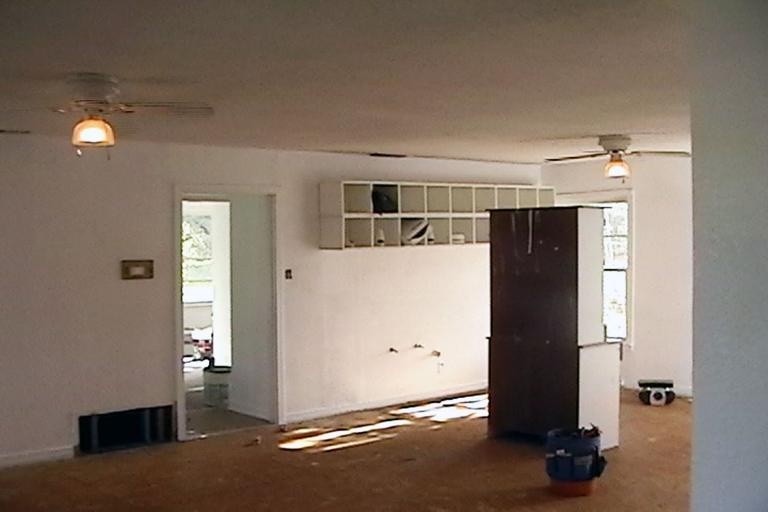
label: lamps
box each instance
[602,154,632,178]
[71,116,116,160]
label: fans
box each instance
[546,135,691,162]
[1,76,216,120]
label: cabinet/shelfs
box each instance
[320,178,553,249]
[485,205,620,452]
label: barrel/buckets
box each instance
[203,365,232,407]
